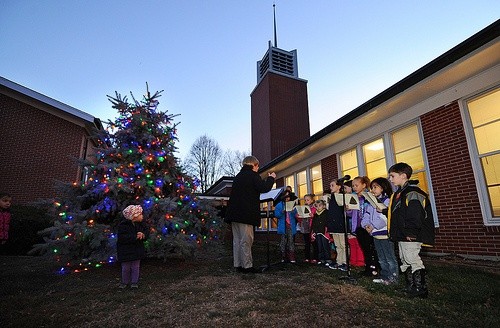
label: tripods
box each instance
[257,187,284,272]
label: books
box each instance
[334,193,360,210]
[286,198,298,211]
[360,192,387,209]
[295,205,311,218]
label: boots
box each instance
[403,268,428,299]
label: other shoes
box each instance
[236,266,255,273]
[374,278,391,285]
[329,263,366,272]
[131,284,138,291]
[116,285,127,293]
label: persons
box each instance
[274,176,400,286]
[376,163,435,299]
[224,156,276,274]
[116,205,150,290]
[0,192,13,245]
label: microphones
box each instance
[337,174,351,184]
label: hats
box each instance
[122,205,142,220]
